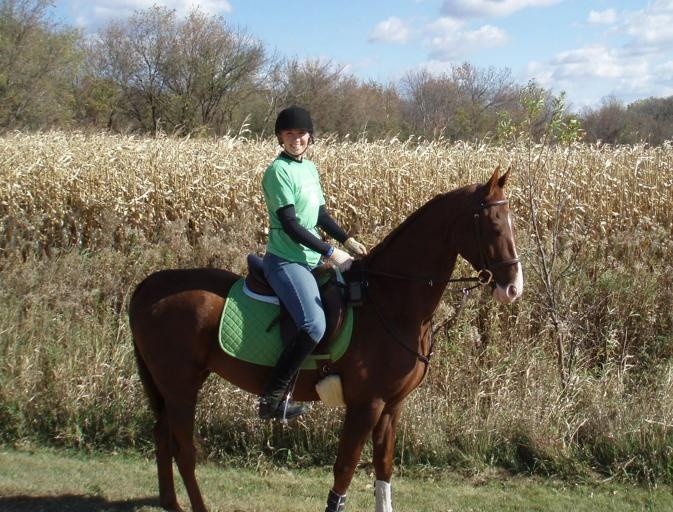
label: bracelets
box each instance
[325,246,334,258]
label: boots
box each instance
[260,329,319,418]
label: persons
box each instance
[257,106,368,420]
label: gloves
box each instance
[344,237,367,255]
[328,248,353,274]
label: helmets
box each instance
[275,107,312,136]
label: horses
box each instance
[126,166,524,512]
